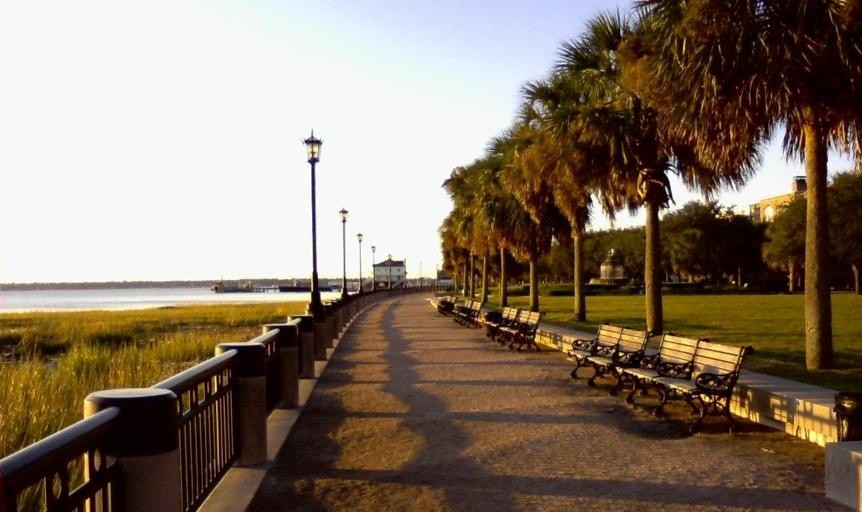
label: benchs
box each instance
[437,295,545,353]
[566,322,754,432]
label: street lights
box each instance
[371,246,375,290]
[356,232,364,293]
[304,129,322,309]
[388,253,391,290]
[339,208,348,295]
